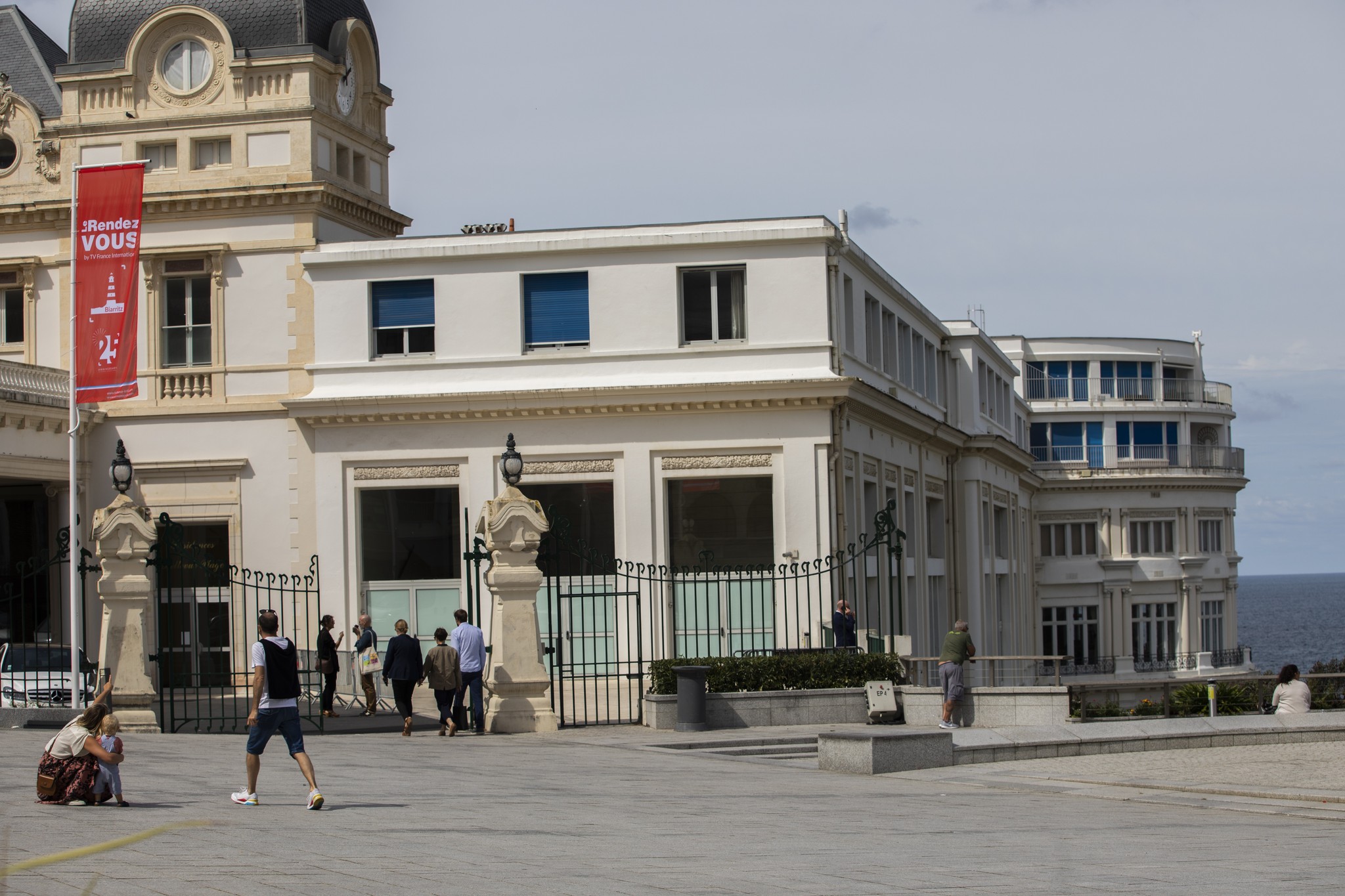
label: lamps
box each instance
[108,439,135,494]
[499,433,524,486]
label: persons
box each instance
[353,614,377,717]
[381,619,424,736]
[938,619,976,728]
[37,674,124,804]
[316,615,344,715]
[419,628,463,737]
[450,608,487,735]
[89,714,130,808]
[832,600,859,654]
[1272,664,1312,715]
[230,609,325,810]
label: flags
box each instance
[75,164,139,403]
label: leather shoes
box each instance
[449,723,457,737]
[439,731,446,736]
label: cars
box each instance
[0,642,97,709]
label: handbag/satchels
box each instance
[315,657,334,674]
[37,773,57,796]
[358,646,383,675]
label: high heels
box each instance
[319,709,326,716]
[325,710,340,718]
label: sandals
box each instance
[404,717,412,734]
[93,800,101,806]
[117,801,130,807]
[402,731,411,736]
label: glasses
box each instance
[259,609,278,619]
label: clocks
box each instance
[337,45,356,117]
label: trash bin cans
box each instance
[670,665,712,732]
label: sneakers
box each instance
[307,788,324,810]
[446,725,451,730]
[939,720,944,727]
[64,798,86,806]
[470,727,485,735]
[359,711,376,717]
[231,786,259,805]
[941,720,959,729]
